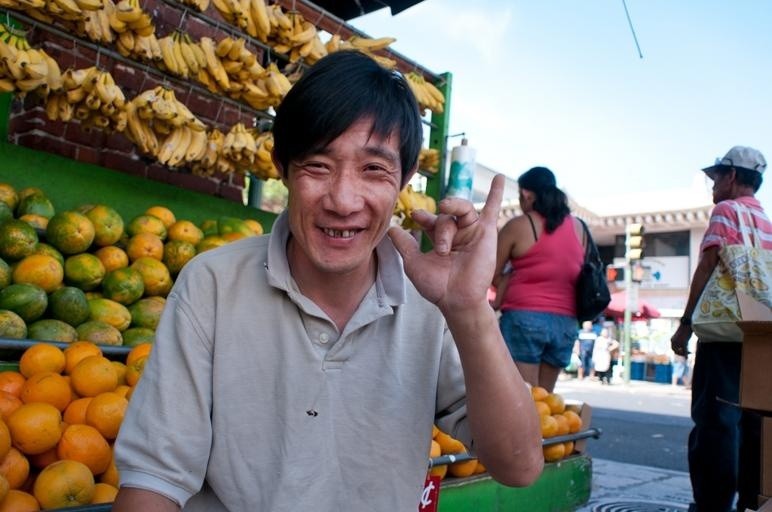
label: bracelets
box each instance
[679,316,692,326]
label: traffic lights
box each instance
[625,222,644,260]
[606,267,624,282]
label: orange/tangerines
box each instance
[524,380,584,463]
[0,183,263,346]
[429,424,486,484]
[0,341,159,512]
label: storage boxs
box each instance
[736,288,772,512]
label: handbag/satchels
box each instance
[692,243,772,342]
[576,262,611,321]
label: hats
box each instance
[702,145,766,180]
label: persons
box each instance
[489,258,514,311]
[672,355,690,387]
[489,166,591,393]
[671,145,772,511]
[591,326,620,386]
[111,49,546,512]
[576,320,597,384]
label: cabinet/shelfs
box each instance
[0,0,603,512]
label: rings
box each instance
[677,347,683,351]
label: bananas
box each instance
[186,1,446,117]
[0,10,281,180]
[418,149,441,175]
[0,0,298,110]
[396,184,439,224]
